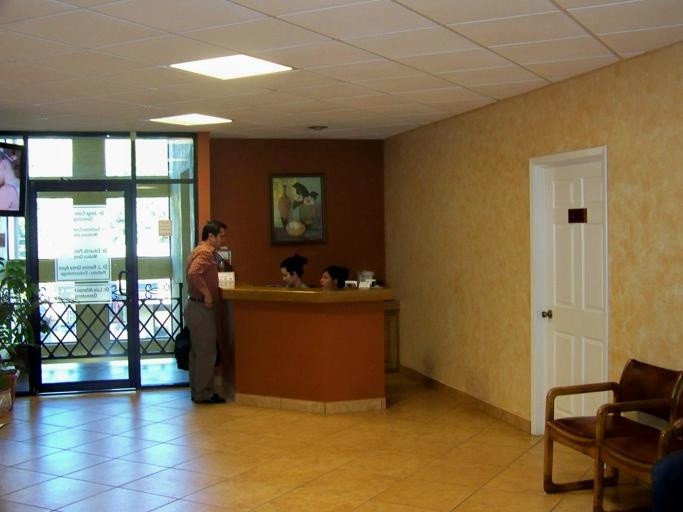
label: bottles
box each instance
[278,185,291,230]
[218,259,225,272]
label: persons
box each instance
[181,218,228,405]
[276,253,310,290]
[1,148,20,211]
[318,265,350,291]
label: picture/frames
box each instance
[271,172,327,246]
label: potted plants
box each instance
[0,258,53,420]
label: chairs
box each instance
[542,358,683,512]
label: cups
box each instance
[362,271,374,281]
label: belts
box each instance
[187,296,203,302]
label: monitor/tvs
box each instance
[0,143,26,216]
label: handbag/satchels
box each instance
[174,327,221,370]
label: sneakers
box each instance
[192,393,225,403]
